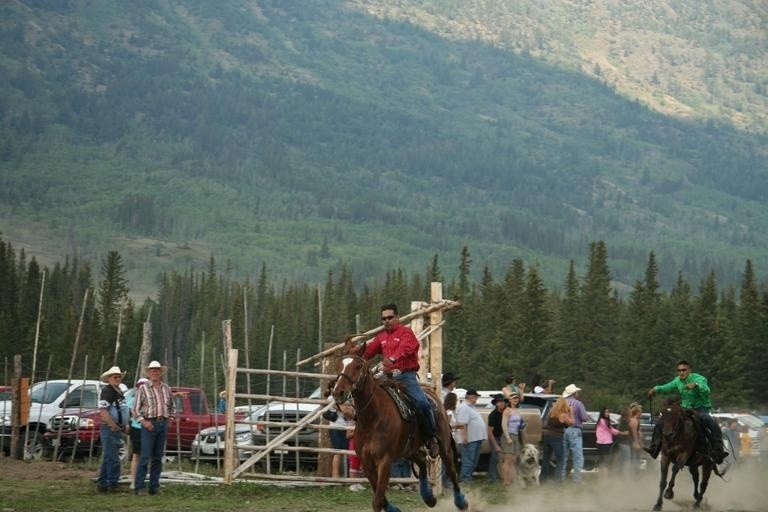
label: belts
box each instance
[146,416,166,422]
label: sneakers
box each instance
[428,439,439,458]
[641,447,658,459]
[358,483,366,489]
[350,485,359,492]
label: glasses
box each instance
[677,369,687,372]
[382,315,394,321]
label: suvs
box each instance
[710,413,767,458]
[0,380,133,462]
[252,385,322,473]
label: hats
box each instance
[562,384,581,397]
[136,378,149,386]
[509,392,519,398]
[100,366,126,383]
[442,372,460,386]
[492,394,508,405]
[143,361,168,374]
[466,388,481,396]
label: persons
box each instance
[219,391,228,414]
[594,407,629,481]
[529,373,555,394]
[128,378,150,490]
[539,398,576,484]
[500,392,529,490]
[642,360,729,464]
[444,392,464,473]
[457,389,486,485]
[488,394,506,485]
[346,413,366,493]
[757,422,768,478]
[363,304,439,458]
[502,376,526,409]
[740,425,756,464]
[97,366,130,493]
[133,361,177,497]
[324,380,356,478]
[615,408,632,474]
[561,384,595,487]
[440,372,460,405]
[725,420,739,465]
[629,406,643,473]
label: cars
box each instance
[190,402,284,461]
[487,393,620,470]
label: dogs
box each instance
[517,444,542,488]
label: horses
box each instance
[332,336,469,512]
[652,397,723,512]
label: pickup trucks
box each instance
[45,388,248,464]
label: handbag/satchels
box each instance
[322,404,338,421]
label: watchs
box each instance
[694,382,698,388]
[388,356,396,363]
